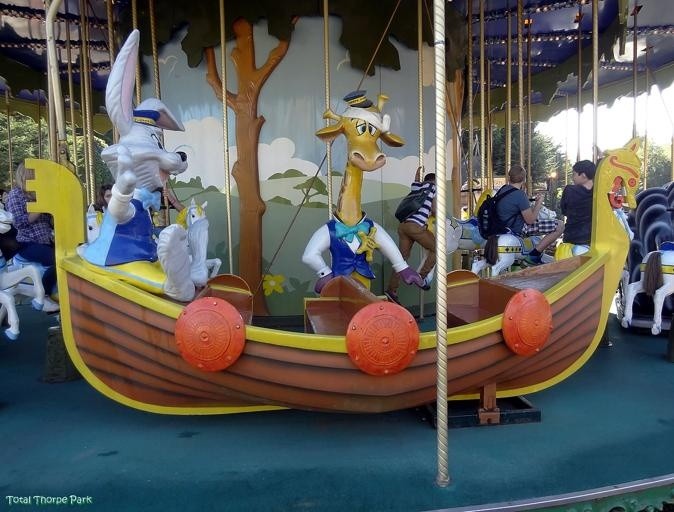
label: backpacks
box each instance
[478,184,519,240]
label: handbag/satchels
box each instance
[0,227,21,261]
[395,187,428,223]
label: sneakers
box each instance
[414,273,431,291]
[383,287,401,305]
[521,252,545,267]
[32,294,59,312]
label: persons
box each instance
[381,164,441,306]
[492,165,562,264]
[9,162,60,314]
[83,185,113,212]
[560,160,596,246]
